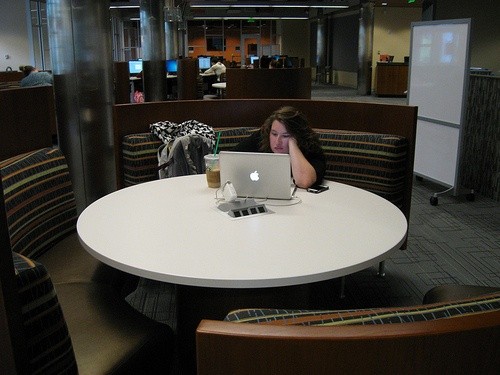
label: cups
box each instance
[204,154,220,188]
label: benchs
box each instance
[122,127,407,207]
[0,145,157,375]
[221,283,500,326]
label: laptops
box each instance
[219,150,296,200]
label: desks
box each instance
[376,61,409,98]
[130,72,226,99]
[76,174,408,300]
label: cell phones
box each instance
[307,185,329,194]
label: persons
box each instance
[268,59,282,68]
[20,65,53,87]
[204,57,227,94]
[235,106,326,189]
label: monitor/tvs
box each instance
[251,56,259,64]
[198,55,212,72]
[166,60,178,75]
[129,59,143,76]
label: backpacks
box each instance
[158,135,214,179]
